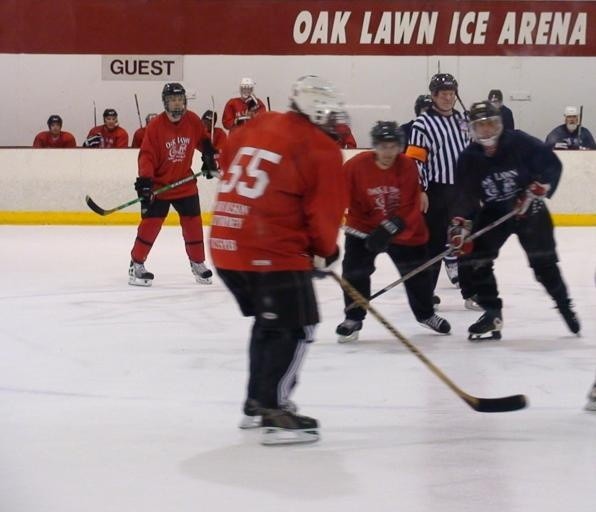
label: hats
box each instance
[563,106,579,116]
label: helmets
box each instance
[369,121,408,156]
[240,77,256,99]
[203,110,217,126]
[47,115,62,128]
[162,82,187,118]
[411,73,503,139]
[103,109,118,125]
[288,75,352,135]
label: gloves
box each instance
[247,96,260,112]
[365,213,405,255]
[201,158,217,179]
[234,116,250,125]
[515,181,551,219]
[447,216,472,257]
[134,177,153,204]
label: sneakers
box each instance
[129,260,154,279]
[336,319,362,336]
[445,262,458,284]
[468,309,503,332]
[420,314,450,333]
[243,398,318,429]
[190,260,212,278]
[556,298,580,333]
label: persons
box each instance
[545,106,595,150]
[84,110,129,147]
[405,95,432,140]
[209,77,345,430]
[488,90,514,129]
[409,75,502,310]
[132,78,358,150]
[445,100,581,341]
[129,83,220,279]
[33,115,75,148]
[335,122,450,333]
